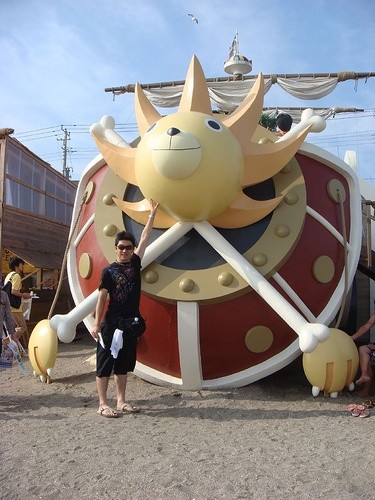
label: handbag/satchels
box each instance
[1,273,22,309]
[119,315,147,341]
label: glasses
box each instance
[116,244,134,250]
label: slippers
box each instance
[345,399,375,418]
[97,403,141,418]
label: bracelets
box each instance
[149,215,155,217]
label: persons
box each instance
[0,257,32,359]
[91,198,159,417]
[350,299,375,397]
[277,113,293,136]
